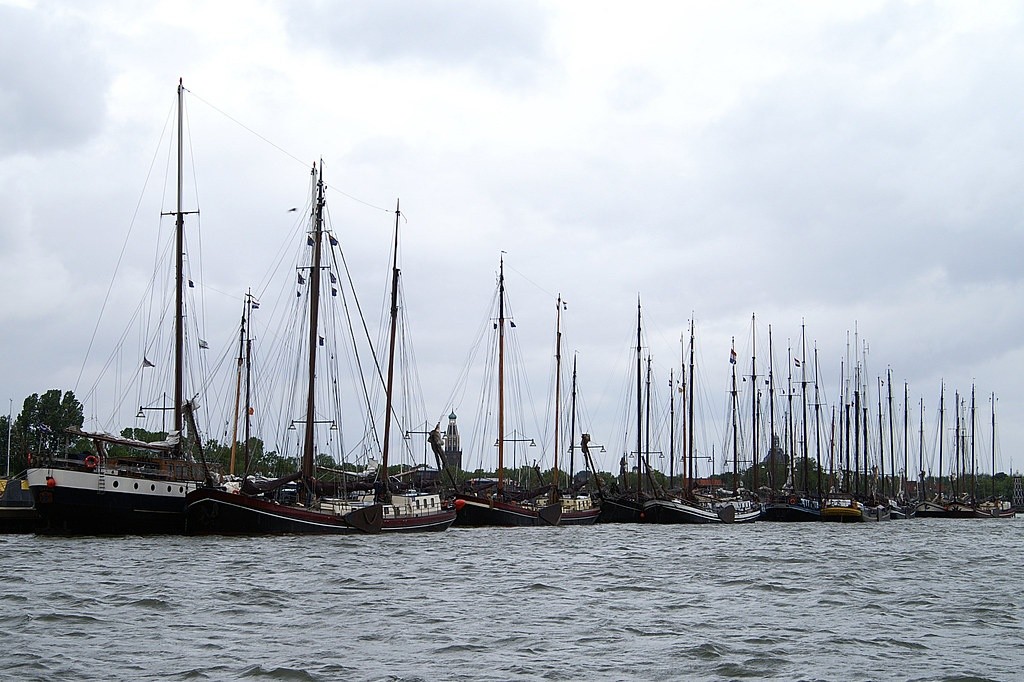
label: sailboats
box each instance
[0,76,1017,538]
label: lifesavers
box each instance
[84,455,97,469]
[790,499,795,505]
